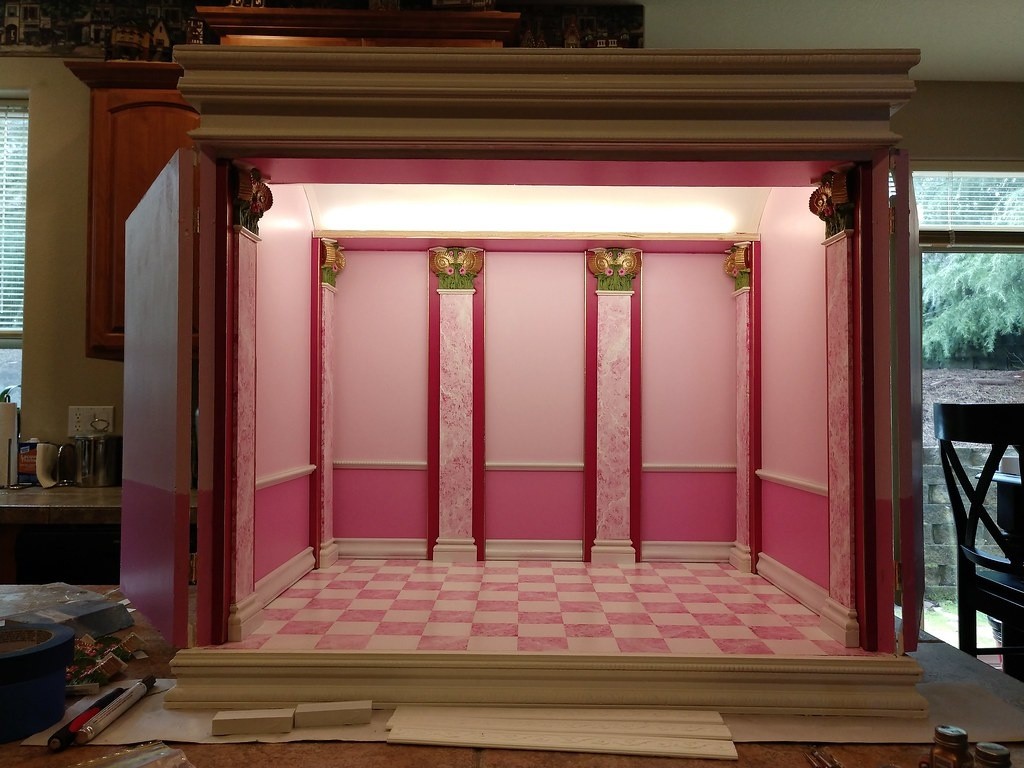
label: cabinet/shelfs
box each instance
[64,6,522,362]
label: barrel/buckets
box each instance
[72,418,122,486]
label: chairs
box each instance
[933,402,1024,680]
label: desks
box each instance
[0,489,199,584]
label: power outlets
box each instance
[66,404,113,439]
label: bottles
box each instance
[930,725,973,768]
[973,742,1011,768]
